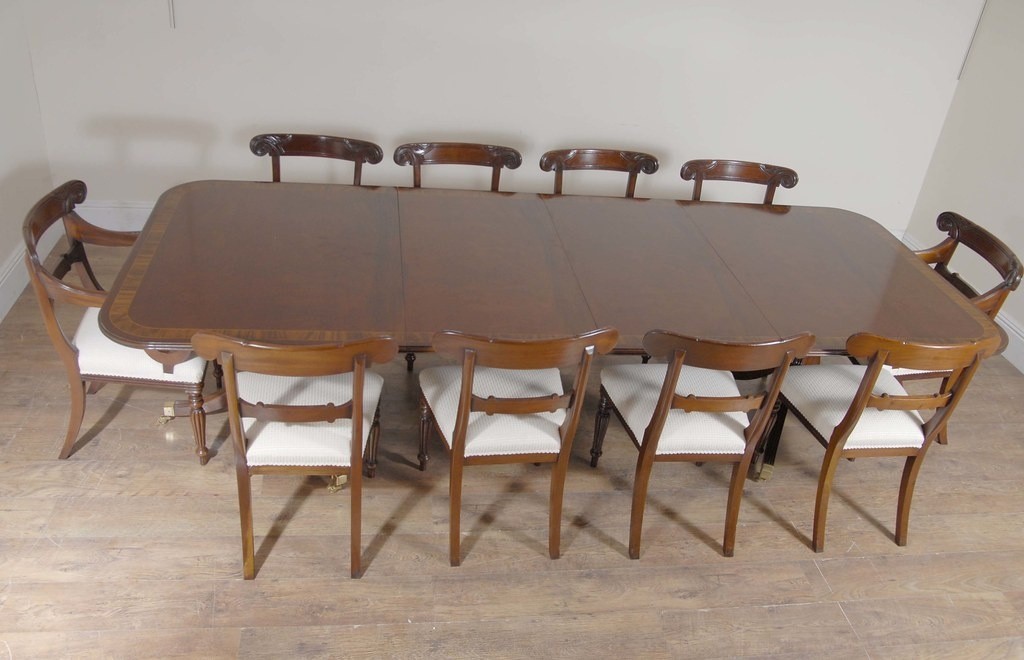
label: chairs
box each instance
[887,212,1023,445]
[207,134,999,580]
[23,180,224,466]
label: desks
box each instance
[97,180,1008,481]
